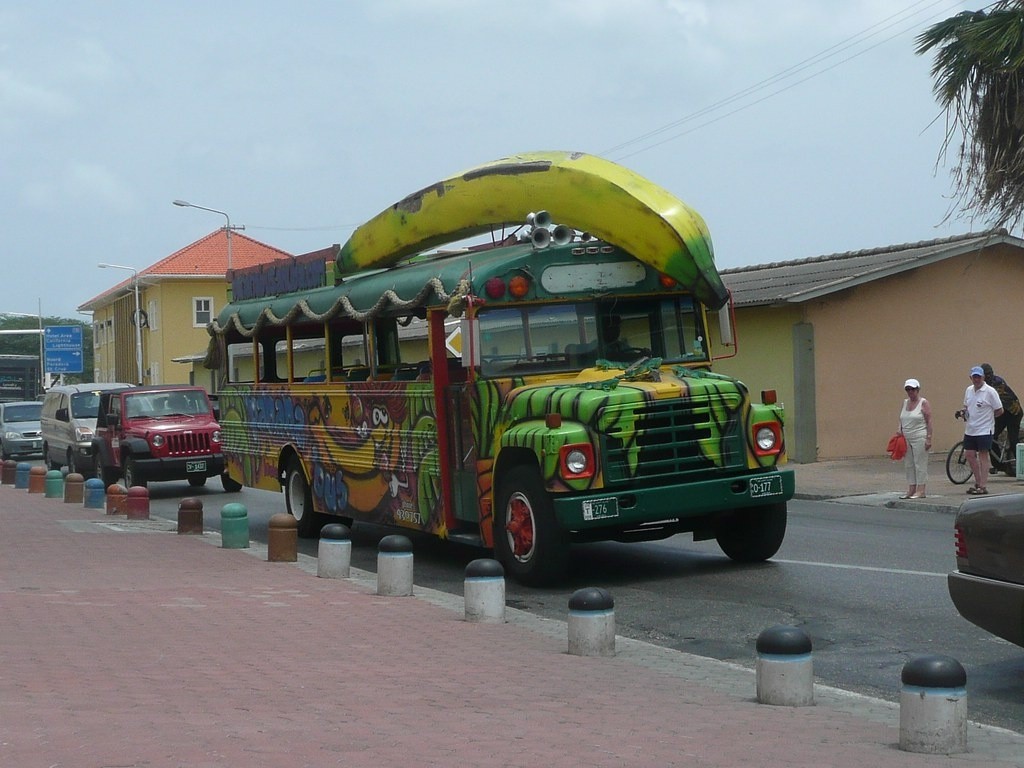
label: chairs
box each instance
[128,399,145,416]
[167,397,187,411]
[563,337,634,369]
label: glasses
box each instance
[905,387,917,391]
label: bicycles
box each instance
[945,408,1024,486]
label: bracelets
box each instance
[928,435,931,438]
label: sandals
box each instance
[966,484,988,494]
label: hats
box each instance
[971,367,984,376]
[905,379,920,388]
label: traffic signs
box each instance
[44,325,84,374]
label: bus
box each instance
[215,150,796,583]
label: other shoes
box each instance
[898,494,914,499]
[909,494,926,498]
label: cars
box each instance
[0,401,44,459]
[947,493,1024,648]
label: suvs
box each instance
[92,384,244,493]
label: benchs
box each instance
[489,355,548,373]
[392,362,462,381]
[345,363,412,381]
[305,364,366,382]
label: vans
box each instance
[40,383,138,475]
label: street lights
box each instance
[172,199,233,270]
[97,262,143,386]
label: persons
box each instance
[585,310,652,360]
[898,378,932,499]
[964,363,1024,494]
